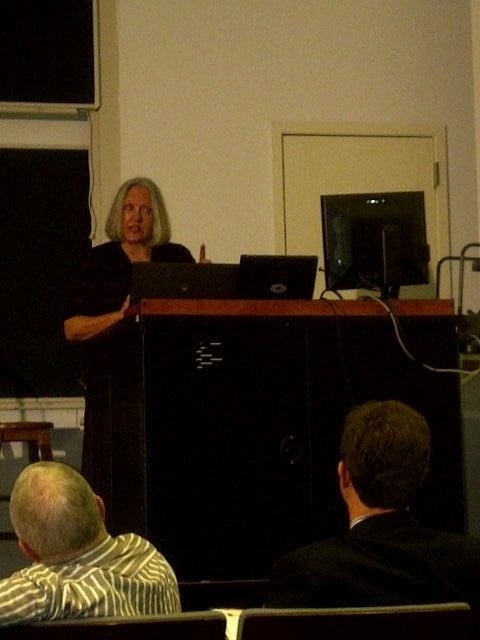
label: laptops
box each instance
[236,255,318,300]
[130,262,240,307]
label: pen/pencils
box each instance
[200,243,205,263]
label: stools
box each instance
[0,422,54,540]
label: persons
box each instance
[262,399,480,612]
[63,178,214,342]
[1,459,183,629]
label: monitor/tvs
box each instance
[321,191,428,299]
